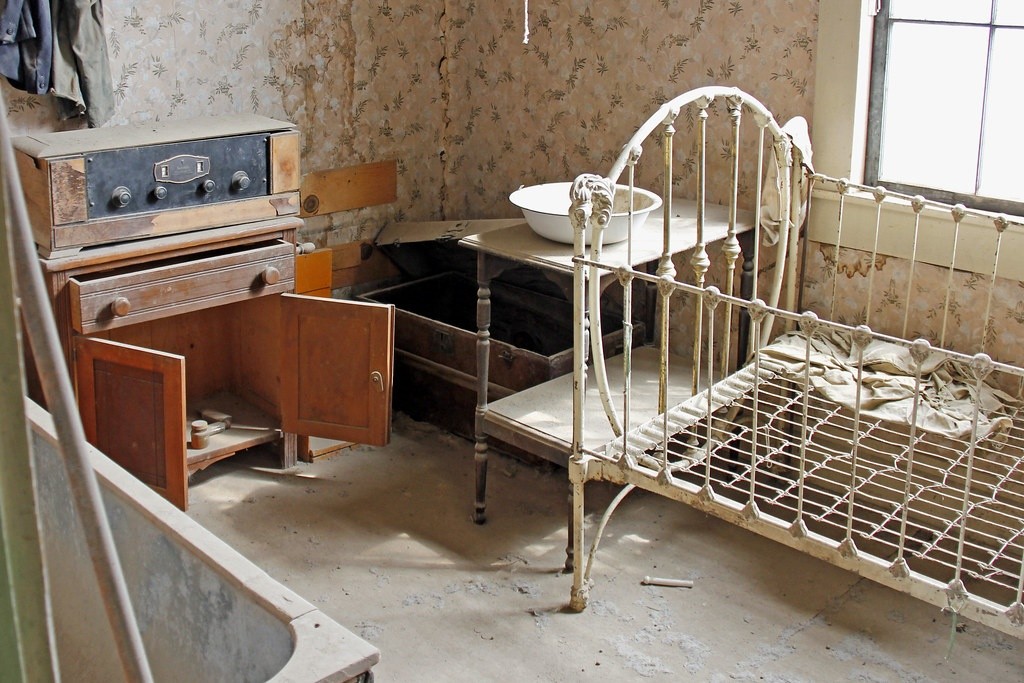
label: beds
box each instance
[566,86,1024,639]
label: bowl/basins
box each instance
[510,184,663,244]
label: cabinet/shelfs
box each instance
[17,216,397,513]
[457,195,761,571]
[351,270,646,468]
